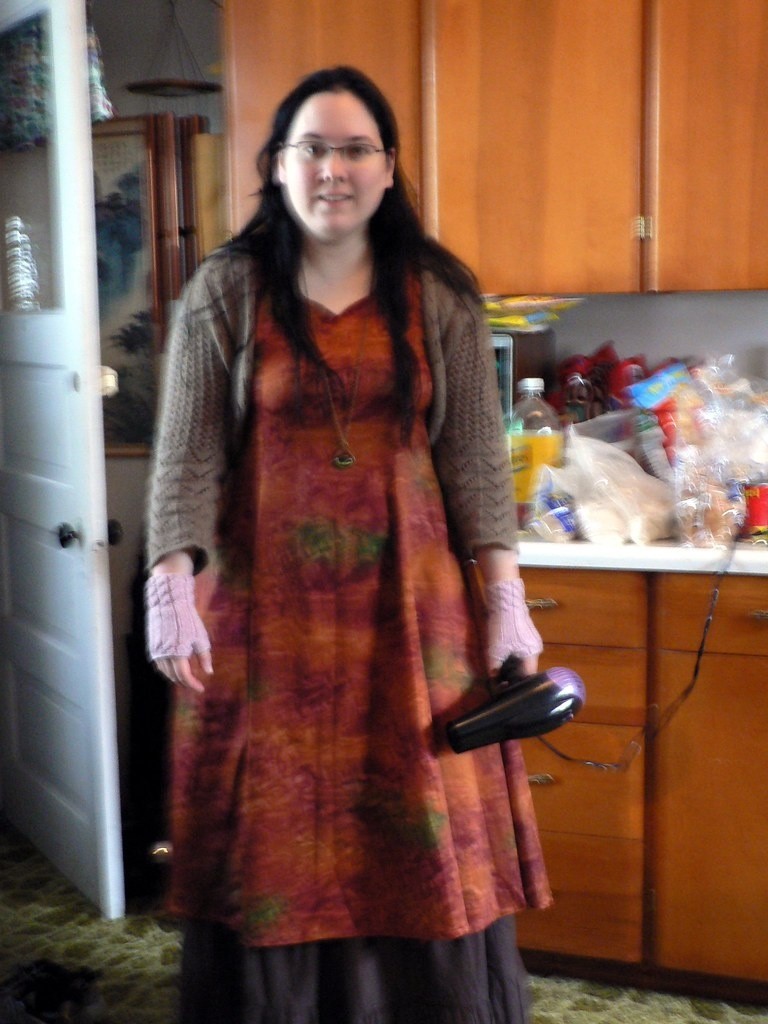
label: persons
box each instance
[147,65,542,1023]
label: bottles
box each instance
[507,378,558,533]
[525,491,581,543]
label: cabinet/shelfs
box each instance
[648,573,768,982]
[515,564,648,963]
[421,0,768,296]
[224,0,421,243]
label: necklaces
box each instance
[294,243,377,470]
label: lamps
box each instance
[124,0,222,98]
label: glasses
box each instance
[280,141,389,164]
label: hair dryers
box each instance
[441,655,585,755]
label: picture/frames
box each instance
[90,111,185,461]
